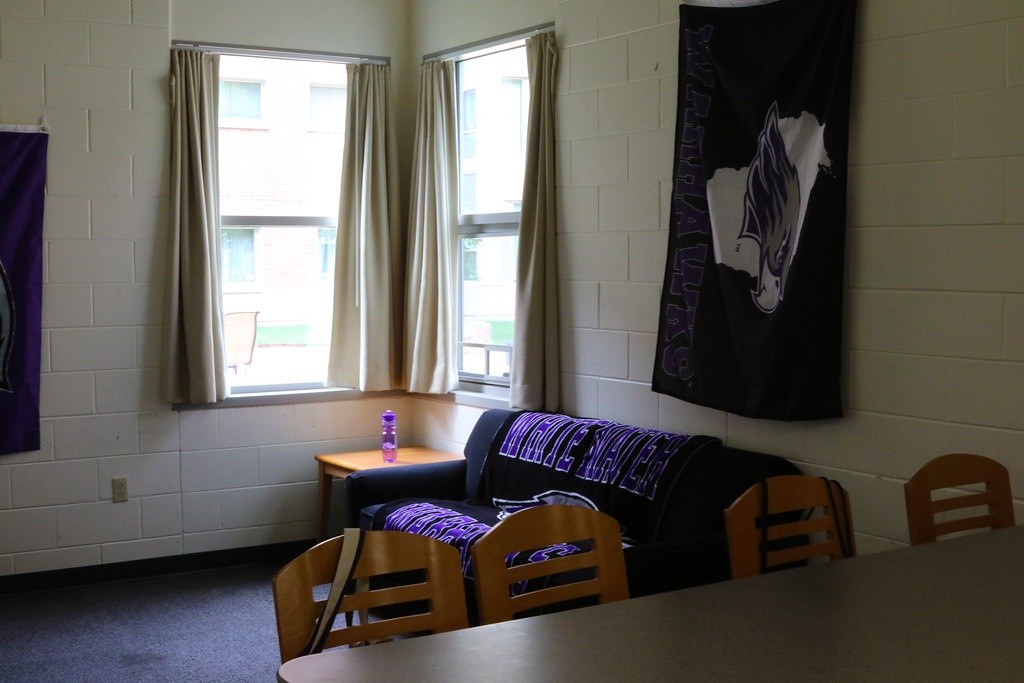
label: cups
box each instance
[382,409,397,462]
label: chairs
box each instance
[724,474,858,581]
[470,505,631,633]
[272,528,468,663]
[904,453,1015,546]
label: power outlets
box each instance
[112,477,128,502]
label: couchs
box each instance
[342,409,811,635]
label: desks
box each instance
[315,445,465,544]
[275,522,1023,683]
